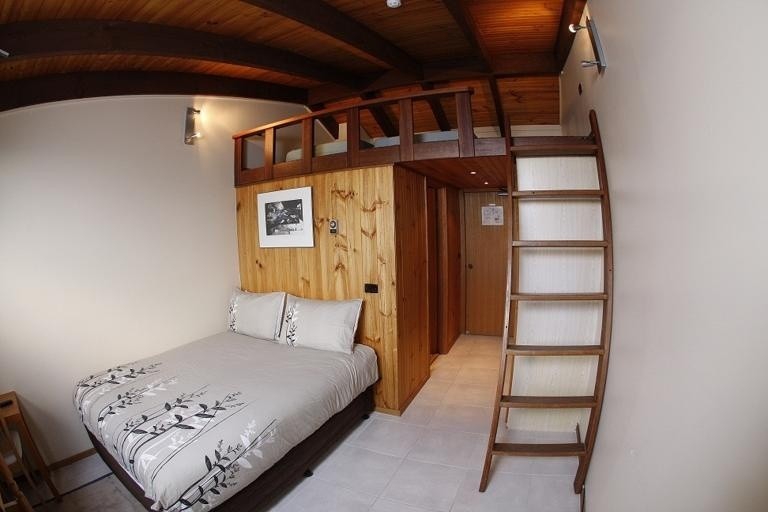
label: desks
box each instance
[0,391,64,504]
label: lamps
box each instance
[568,16,606,75]
[184,107,201,145]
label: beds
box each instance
[77,331,374,512]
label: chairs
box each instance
[0,415,45,509]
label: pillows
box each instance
[279,293,364,355]
[226,288,286,344]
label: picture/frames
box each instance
[256,186,315,249]
